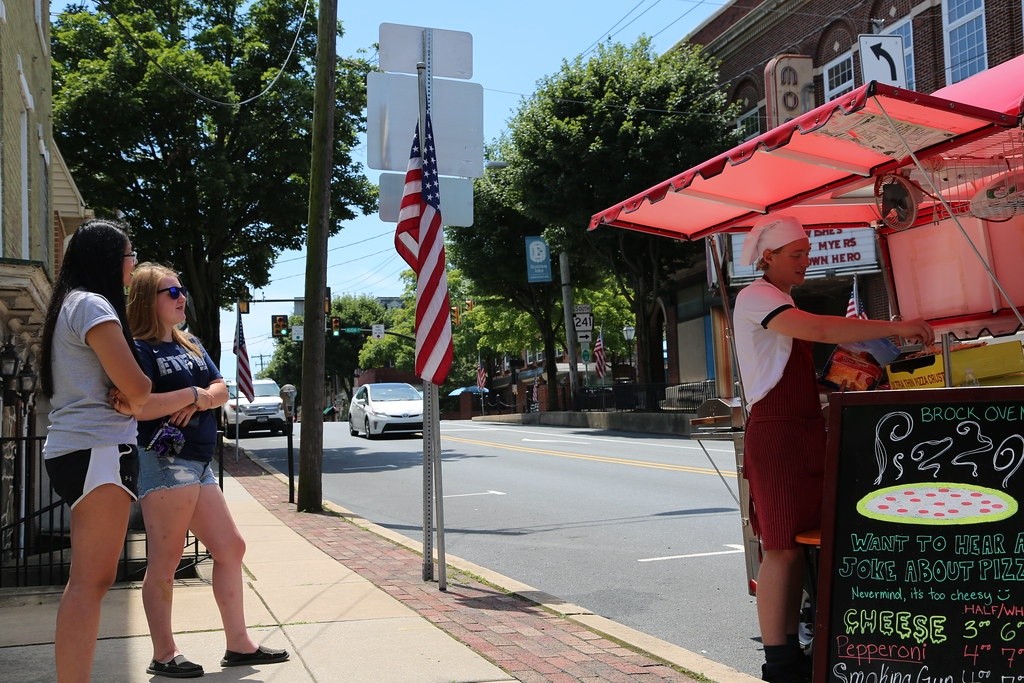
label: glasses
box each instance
[124,251,137,264]
[157,286,187,300]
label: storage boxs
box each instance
[885,340,1024,391]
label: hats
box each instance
[741,214,808,266]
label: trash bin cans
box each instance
[612,364,637,412]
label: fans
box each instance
[873,168,924,232]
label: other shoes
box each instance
[760,648,812,683]
[146,654,205,678]
[222,644,289,667]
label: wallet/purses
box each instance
[152,418,185,457]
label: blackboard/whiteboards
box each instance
[808,384,1023,683]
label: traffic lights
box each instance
[332,315,340,336]
[272,314,288,338]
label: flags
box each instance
[477,366,487,389]
[532,379,538,403]
[846,286,868,319]
[593,334,607,379]
[233,306,254,403]
[391,81,454,386]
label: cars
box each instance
[217,376,286,440]
[346,383,424,442]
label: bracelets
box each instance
[191,386,199,404]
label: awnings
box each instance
[322,406,333,414]
[588,79,1024,424]
[448,385,489,396]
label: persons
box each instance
[127,261,291,678]
[38,219,152,683]
[732,212,935,683]
